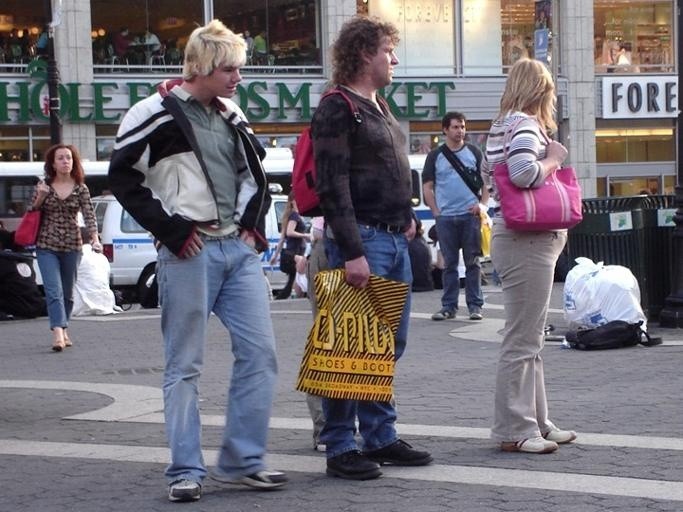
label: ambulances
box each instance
[83,181,311,307]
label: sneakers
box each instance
[208,469,290,488]
[432,304,456,320]
[52,340,72,350]
[168,479,201,502]
[500,426,577,454]
[469,303,482,320]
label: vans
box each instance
[402,153,494,269]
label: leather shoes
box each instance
[317,439,434,481]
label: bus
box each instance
[0,147,295,232]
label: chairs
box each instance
[8,42,276,73]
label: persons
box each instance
[70,226,123,318]
[421,108,489,321]
[29,144,105,351]
[535,9,548,29]
[474,57,582,455]
[106,18,286,501]
[1,18,48,73]
[243,28,315,73]
[605,45,630,73]
[270,14,435,483]
[94,26,187,72]
[410,220,467,292]
[269,191,325,300]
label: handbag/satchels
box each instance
[494,164,583,232]
[14,210,41,246]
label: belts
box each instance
[355,213,404,234]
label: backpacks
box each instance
[291,88,359,217]
[566,320,662,350]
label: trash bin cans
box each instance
[567,195,681,326]
